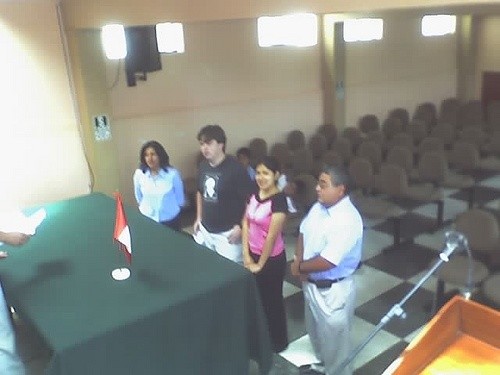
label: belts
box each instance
[308,277,346,284]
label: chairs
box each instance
[184,100,500,317]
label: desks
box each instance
[0,192,274,375]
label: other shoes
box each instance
[299,363,325,375]
[272,341,288,353]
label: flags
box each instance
[113,191,134,265]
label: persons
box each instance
[236,147,296,213]
[0,231,29,259]
[192,125,252,262]
[132,141,186,230]
[292,166,364,375]
[240,156,287,352]
